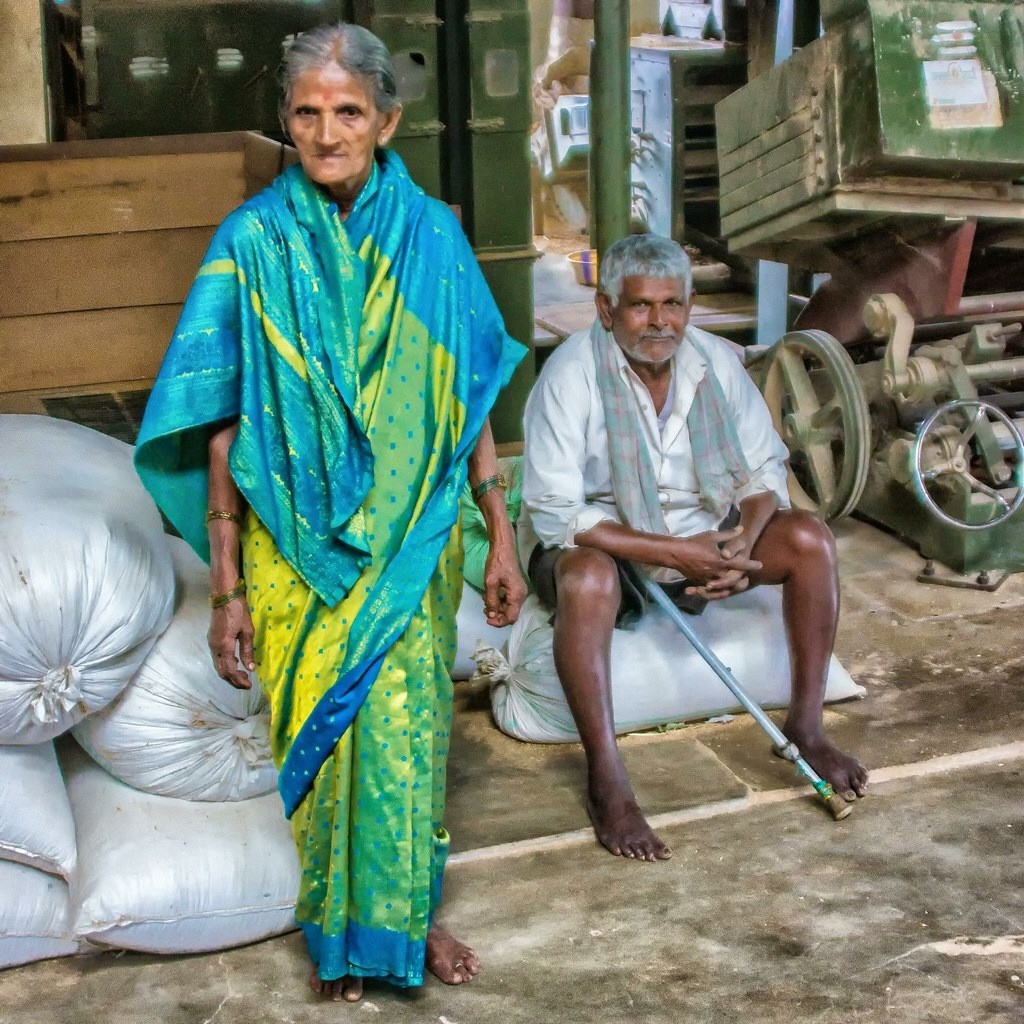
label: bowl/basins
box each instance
[566,249,597,286]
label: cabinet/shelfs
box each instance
[589,32,747,242]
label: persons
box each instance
[516,237,867,863]
[133,25,529,1002]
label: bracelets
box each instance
[208,577,247,608]
[202,511,244,527]
[470,475,508,505]
